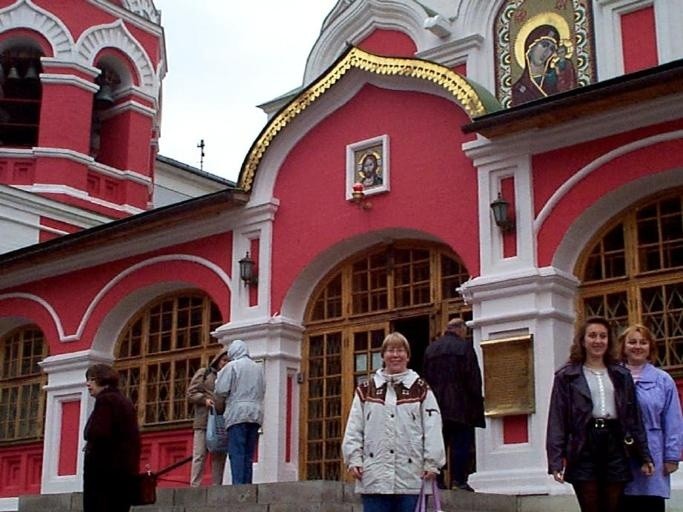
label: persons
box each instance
[182,350,229,486]
[513,22,558,104]
[214,338,265,483]
[543,315,654,510]
[338,330,446,509]
[423,316,486,492]
[357,153,382,188]
[615,321,682,511]
[555,46,578,95]
[81,364,141,512]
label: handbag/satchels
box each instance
[205,404,228,454]
[130,470,159,506]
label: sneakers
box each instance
[437,481,475,492]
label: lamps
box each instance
[489,191,517,236]
[238,251,257,287]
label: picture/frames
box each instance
[344,134,391,202]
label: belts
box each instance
[588,417,621,428]
